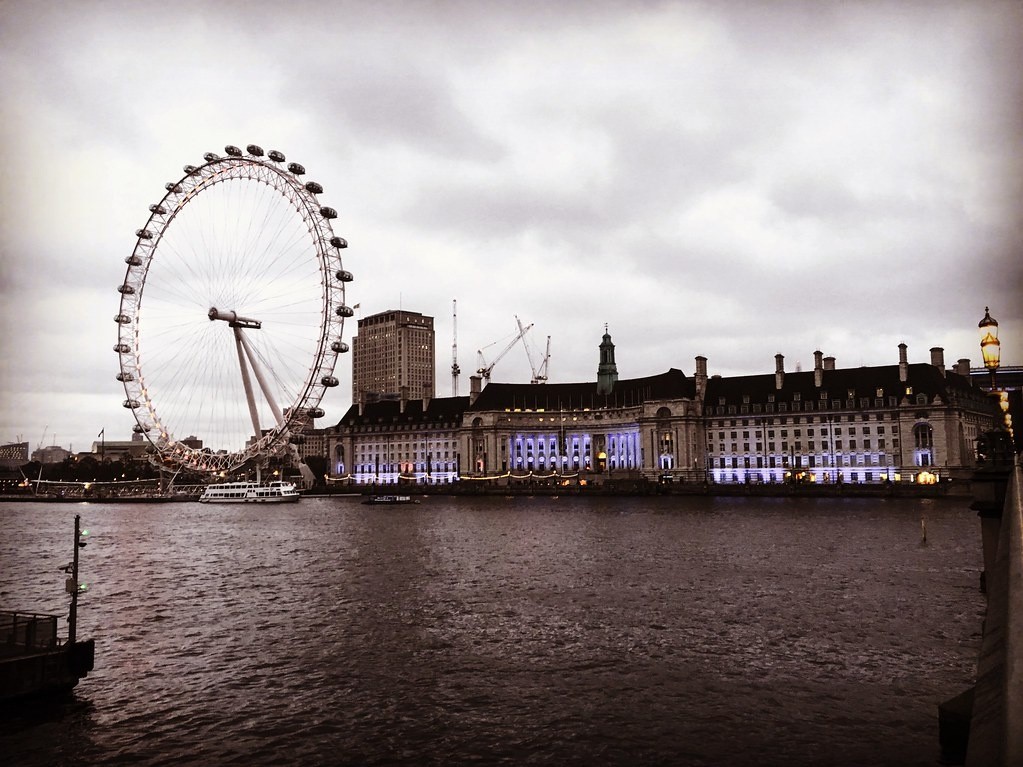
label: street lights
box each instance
[703,422,708,482]
[386,434,390,473]
[761,418,768,468]
[896,410,903,467]
[424,432,429,483]
[979,306,1003,430]
[828,416,835,484]
[999,386,1014,443]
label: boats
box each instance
[361,495,421,504]
[198,481,300,502]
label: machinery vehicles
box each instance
[451,300,551,397]
